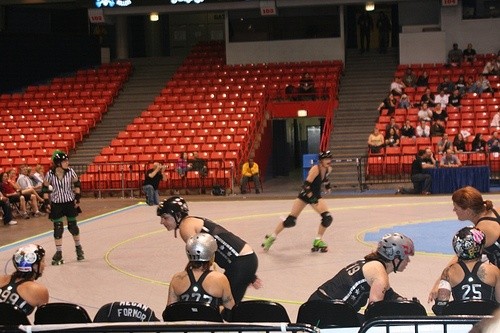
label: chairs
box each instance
[0,299,500,333]
[367,52,500,177]
[0,41,343,190]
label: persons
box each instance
[261,151,334,252]
[177,150,208,180]
[157,196,263,305]
[241,157,261,194]
[427,185,500,304]
[365,43,500,196]
[142,161,169,206]
[297,72,316,100]
[41,149,86,265]
[295,232,415,329]
[166,233,237,324]
[0,164,53,225]
[0,243,49,317]
[431,226,500,316]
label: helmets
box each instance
[379,233,414,259]
[12,244,46,274]
[451,227,486,260]
[185,233,218,262]
[157,196,188,217]
[319,150,332,160]
[53,153,70,165]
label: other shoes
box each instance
[8,220,17,225]
[40,205,51,213]
[39,199,51,204]
[22,213,29,218]
[147,200,153,206]
[34,210,45,217]
[256,189,260,194]
[423,190,431,195]
[241,190,246,193]
[395,188,403,194]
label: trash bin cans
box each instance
[303,153,320,180]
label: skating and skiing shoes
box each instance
[52,251,64,265]
[311,239,328,252]
[75,245,84,260]
[262,234,276,251]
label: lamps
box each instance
[365,0,375,11]
[150,0,159,21]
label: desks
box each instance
[423,165,489,194]
